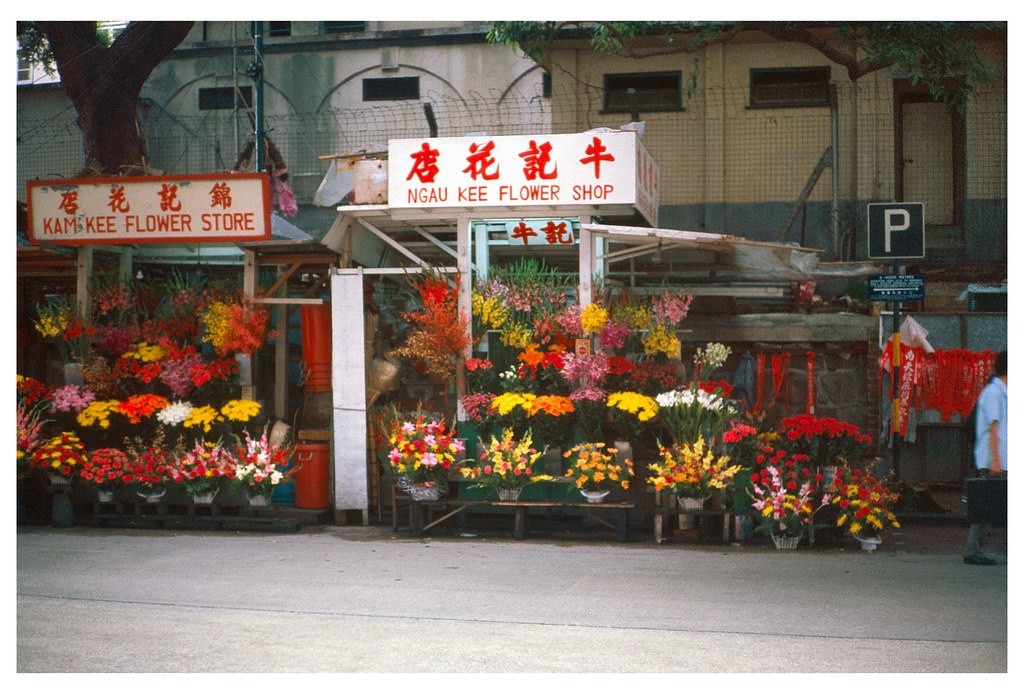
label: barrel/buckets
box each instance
[269,442,330,510]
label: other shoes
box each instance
[964,555,997,566]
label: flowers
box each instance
[16,276,894,533]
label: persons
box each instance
[961,351,1008,566]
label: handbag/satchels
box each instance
[962,472,1007,523]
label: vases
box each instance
[399,479,445,501]
[769,535,881,551]
[187,490,217,503]
[96,490,115,500]
[497,488,519,501]
[247,494,271,506]
[675,498,705,510]
[136,491,166,503]
[582,488,610,503]
[50,507,74,527]
[47,474,73,485]
